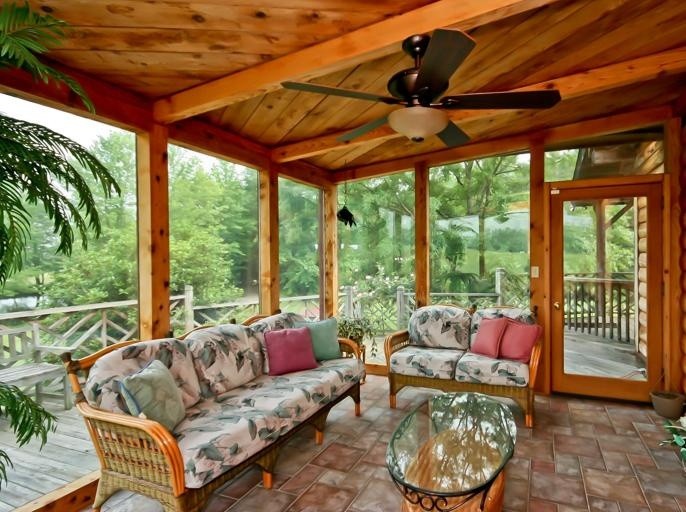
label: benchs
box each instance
[384,302,543,434]
[1,322,77,413]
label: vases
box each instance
[649,391,686,418]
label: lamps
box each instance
[385,95,448,144]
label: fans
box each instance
[281,28,561,149]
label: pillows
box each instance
[468,312,509,358]
[263,327,320,374]
[292,316,344,363]
[496,315,546,364]
[123,357,187,436]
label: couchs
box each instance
[61,311,367,512]
